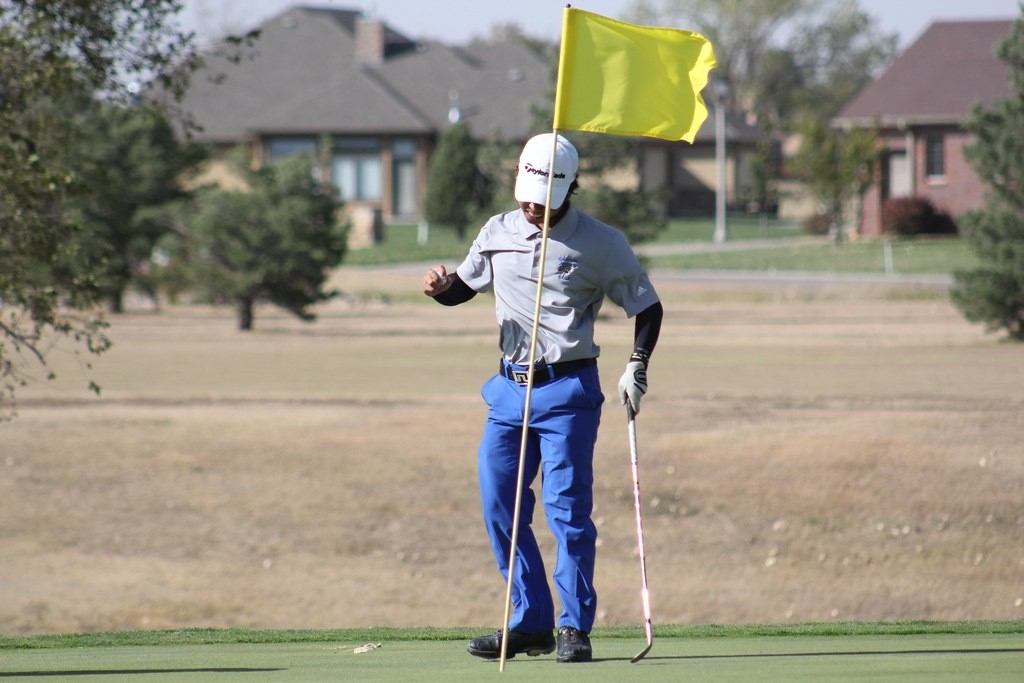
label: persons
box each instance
[423,133,664,663]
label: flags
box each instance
[553,5,717,144]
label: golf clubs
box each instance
[626,397,654,663]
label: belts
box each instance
[499,357,597,385]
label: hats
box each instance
[514,132,579,210]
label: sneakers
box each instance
[467,629,557,659]
[556,626,592,662]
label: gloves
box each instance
[618,362,648,416]
